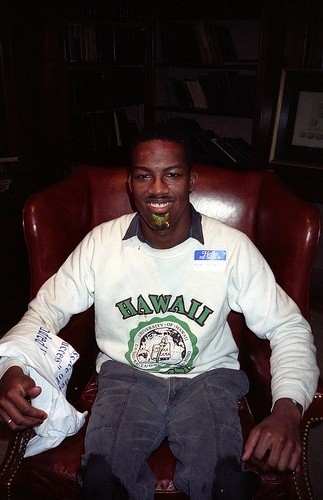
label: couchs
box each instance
[0,164,323,500]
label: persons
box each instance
[0,130,322,500]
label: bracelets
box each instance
[288,397,302,407]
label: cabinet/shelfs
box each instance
[147,0,288,171]
[37,0,160,177]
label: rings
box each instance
[6,418,13,426]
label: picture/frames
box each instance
[270,69,323,169]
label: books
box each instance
[65,0,262,166]
